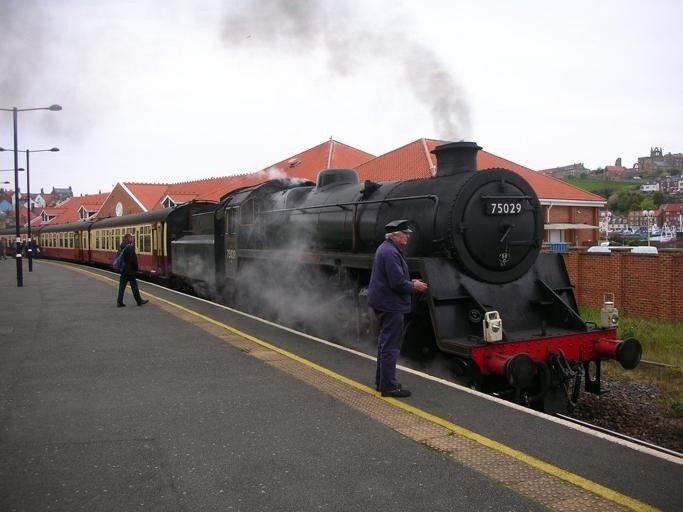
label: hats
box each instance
[125,233,136,238]
[384,220,414,233]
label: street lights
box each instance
[643,210,655,246]
[1,104,62,286]
[600,211,612,242]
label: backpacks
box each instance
[113,243,131,274]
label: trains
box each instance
[2,142,642,414]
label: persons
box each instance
[365,219,427,398]
[116,233,149,306]
[0,237,40,261]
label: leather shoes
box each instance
[137,300,149,306]
[117,303,126,307]
[377,381,412,398]
